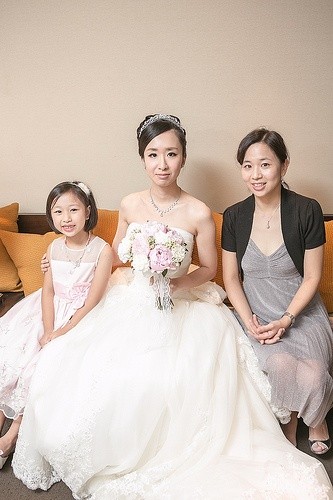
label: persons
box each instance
[222,128,333,454]
[0,181,114,470]
[10,114,333,500]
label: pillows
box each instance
[0,202,23,293]
[190,211,241,304]
[93,208,130,272]
[0,229,63,298]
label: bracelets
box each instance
[281,312,296,328]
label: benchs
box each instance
[0,214,333,325]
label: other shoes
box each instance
[0,456,8,469]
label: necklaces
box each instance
[149,187,182,217]
[66,231,90,273]
[267,206,279,229]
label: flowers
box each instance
[116,221,187,311]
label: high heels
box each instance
[307,420,331,456]
[283,421,299,450]
